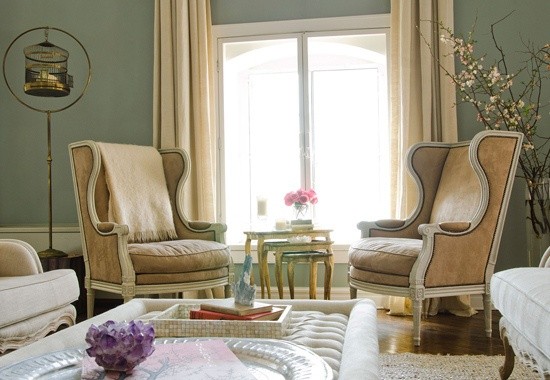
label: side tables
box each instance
[38,255,83,312]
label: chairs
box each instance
[348,131,524,347]
[67,138,232,321]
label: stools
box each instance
[272,251,333,300]
[260,240,335,298]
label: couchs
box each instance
[0,238,80,354]
[489,244,550,380]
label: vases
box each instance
[524,177,550,267]
[292,206,307,220]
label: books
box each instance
[188,304,286,323]
[202,296,272,317]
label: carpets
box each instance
[379,352,550,379]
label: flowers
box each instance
[284,189,319,212]
[416,6,549,238]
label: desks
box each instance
[9,296,379,379]
[244,229,334,302]
